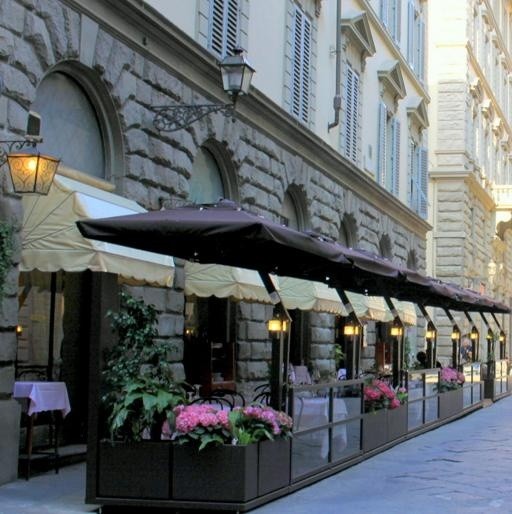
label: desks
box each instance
[14,381,72,481]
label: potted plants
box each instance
[97,295,195,500]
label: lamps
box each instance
[470,332,478,340]
[267,317,287,333]
[343,323,359,336]
[426,329,437,338]
[453,330,460,339]
[151,45,257,133]
[391,326,404,337]
[1,139,63,197]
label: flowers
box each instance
[274,408,294,434]
[160,400,278,451]
[364,380,407,409]
[439,367,465,392]
